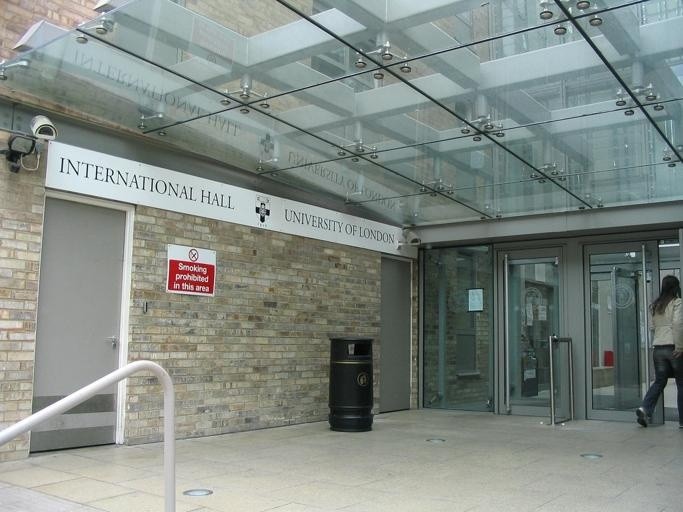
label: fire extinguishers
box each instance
[522,349,538,396]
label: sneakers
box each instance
[636,407,649,428]
[679,424,683,428]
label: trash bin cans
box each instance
[329,336,373,430]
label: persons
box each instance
[634,274,682,428]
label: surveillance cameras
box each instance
[30,115,59,141]
[406,232,421,245]
